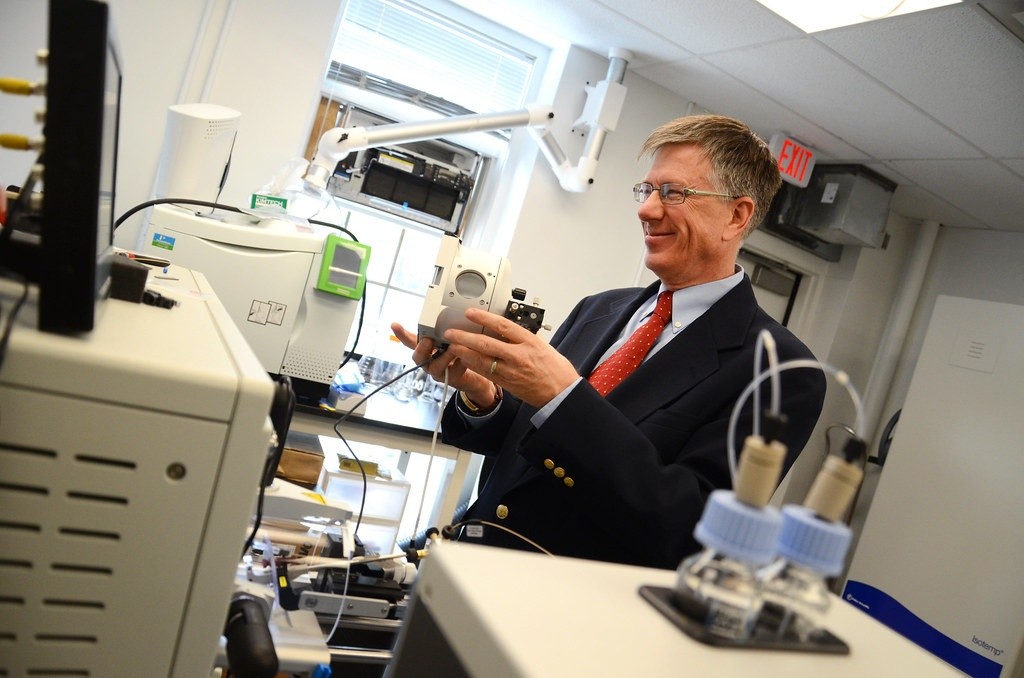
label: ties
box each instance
[586,290,675,399]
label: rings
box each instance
[489,358,498,375]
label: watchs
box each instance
[460,382,503,415]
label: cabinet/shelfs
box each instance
[241,373,471,678]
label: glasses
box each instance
[633,181,740,206]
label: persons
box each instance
[391,114,828,573]
